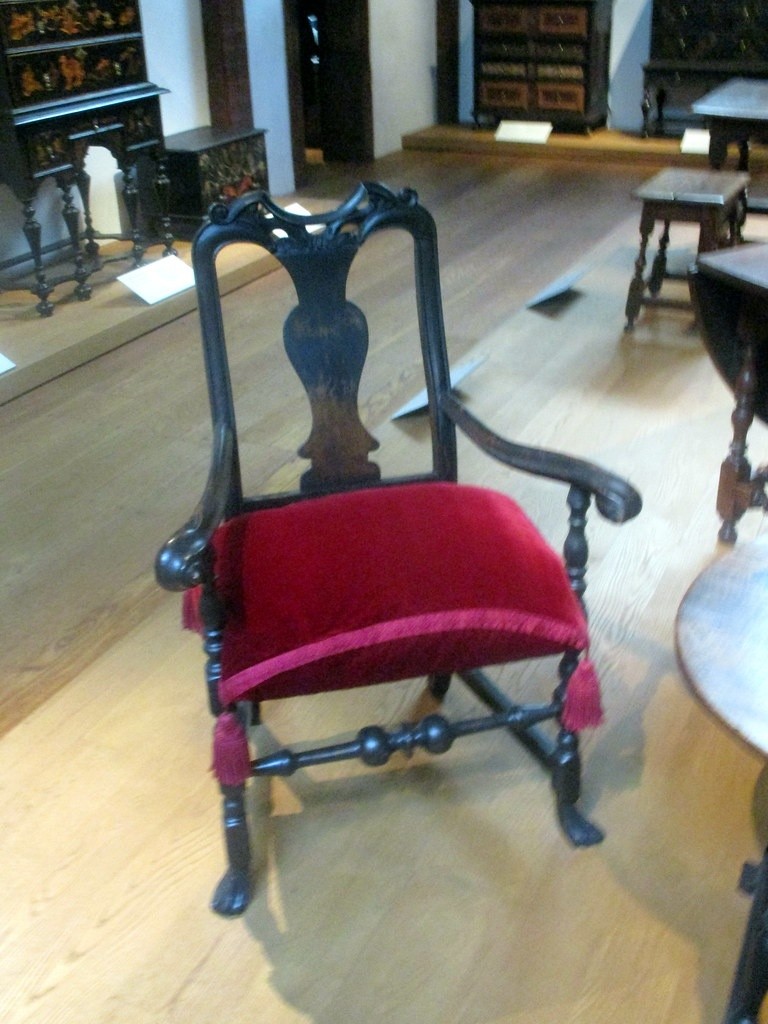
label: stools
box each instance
[624,166,752,337]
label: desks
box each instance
[691,242,768,543]
[690,78,768,214]
[675,530,768,1024]
[162,125,270,243]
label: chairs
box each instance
[155,180,642,917]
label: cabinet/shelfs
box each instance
[0,0,177,319]
[469,0,613,136]
[639,0,768,139]
